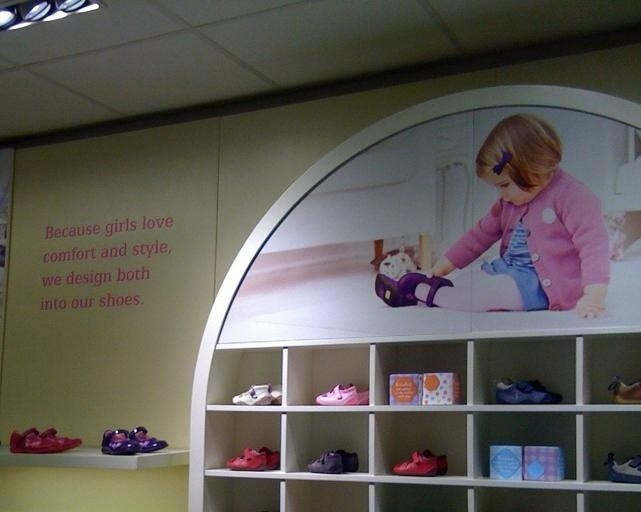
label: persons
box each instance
[374,112,611,318]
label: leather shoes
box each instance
[102,429,137,455]
[129,427,166,452]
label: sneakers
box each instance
[315,384,369,405]
[227,446,280,470]
[608,375,641,404]
[604,452,641,484]
[393,450,447,475]
[496,377,562,404]
[10,427,81,452]
[374,273,417,307]
[308,450,358,473]
[232,383,282,405]
[397,274,453,307]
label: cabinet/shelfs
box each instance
[183,327,641,510]
